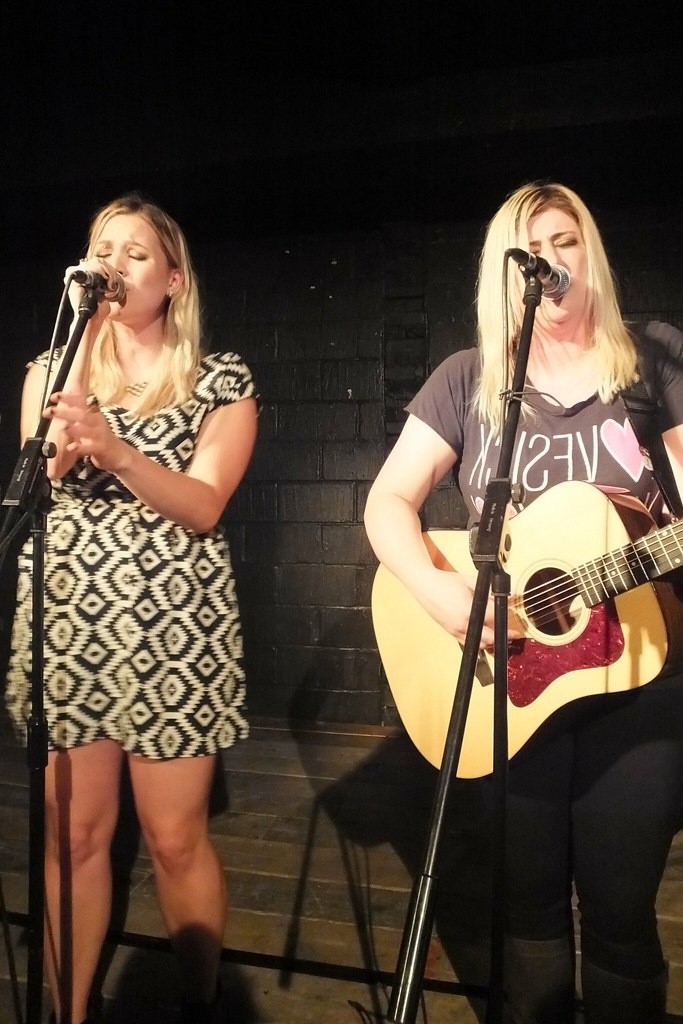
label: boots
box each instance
[585,960,671,1024]
[498,934,574,1024]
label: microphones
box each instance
[505,248,572,300]
[71,270,125,302]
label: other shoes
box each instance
[181,973,223,1024]
[47,990,103,1024]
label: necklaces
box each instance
[122,382,148,397]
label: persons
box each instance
[5,194,257,1024]
[363,180,683,1024]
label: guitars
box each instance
[370,479,683,781]
